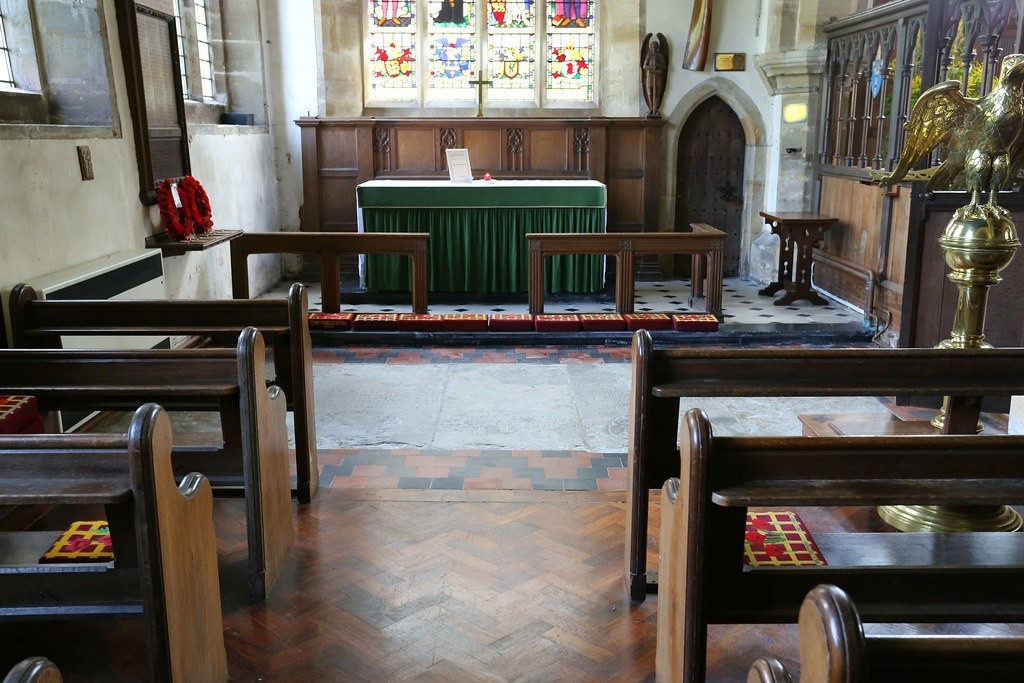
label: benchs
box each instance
[0,280,321,683]
[625,314,1024,683]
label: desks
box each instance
[356,180,607,291]
[759,212,839,305]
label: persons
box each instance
[642,41,666,115]
